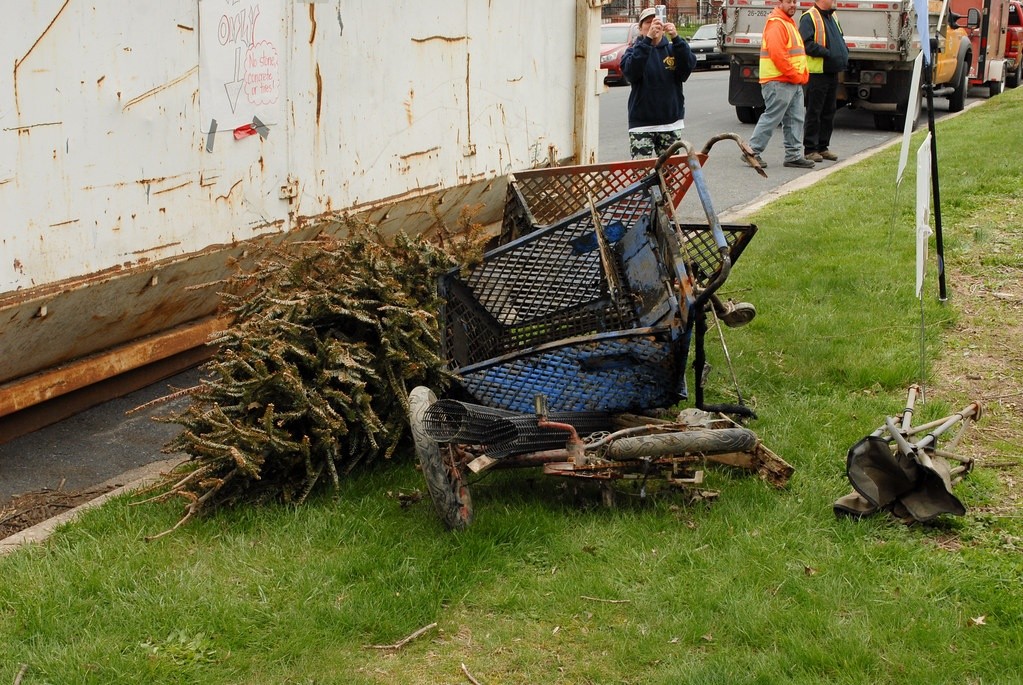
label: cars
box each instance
[685,24,726,72]
[600,22,640,87]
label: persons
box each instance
[797,0,850,161]
[740,0,816,169]
[619,7,697,205]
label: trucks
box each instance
[716,0,1023,134]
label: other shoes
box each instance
[783,157,815,169]
[804,151,824,161]
[741,153,766,168]
[820,149,839,160]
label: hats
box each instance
[637,8,656,27]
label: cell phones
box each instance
[655,5,666,25]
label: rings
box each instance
[670,28,673,31]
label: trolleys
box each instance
[405,129,768,537]
